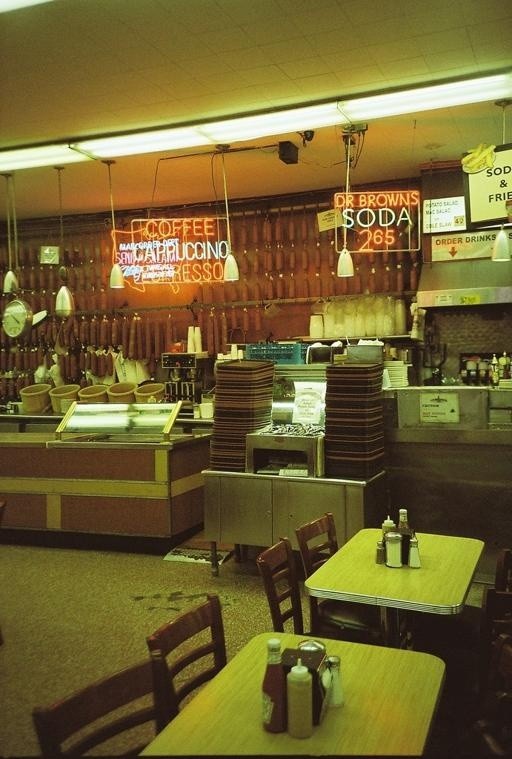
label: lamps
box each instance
[1,173,20,293]
[53,166,75,318]
[215,144,240,281]
[337,135,355,277]
[491,99,512,262]
[101,160,125,289]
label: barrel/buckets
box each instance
[20,382,52,412]
[107,382,138,403]
[77,385,110,403]
[48,385,80,412]
[135,384,165,404]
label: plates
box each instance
[383,360,409,388]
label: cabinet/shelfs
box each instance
[201,467,387,577]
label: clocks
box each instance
[2,300,33,339]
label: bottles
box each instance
[375,509,422,569]
[261,638,345,738]
[491,353,499,386]
